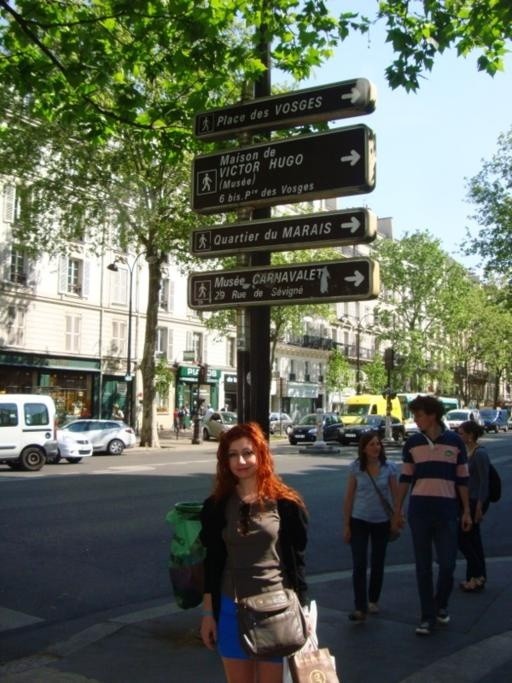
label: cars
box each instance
[268,410,292,437]
[59,419,137,456]
[202,410,237,440]
[289,392,512,446]
[45,424,93,463]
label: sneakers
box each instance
[435,606,451,625]
[463,576,486,591]
[368,601,380,615]
[348,611,366,622]
[415,622,434,635]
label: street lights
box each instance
[338,312,380,394]
[106,250,159,425]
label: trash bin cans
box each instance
[167,502,208,610]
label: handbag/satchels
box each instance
[281,599,320,683]
[286,630,340,683]
[235,588,312,660]
[384,499,400,543]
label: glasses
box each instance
[237,502,252,537]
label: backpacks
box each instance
[470,446,502,503]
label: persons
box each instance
[206,405,213,417]
[112,404,125,419]
[459,420,491,591]
[390,397,473,635]
[200,423,308,682]
[178,405,186,429]
[221,403,229,411]
[342,430,405,619]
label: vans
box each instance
[0,393,58,470]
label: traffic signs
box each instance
[192,205,378,257]
[188,258,380,308]
[193,77,378,143]
[192,124,379,214]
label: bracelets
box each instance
[200,612,215,617]
